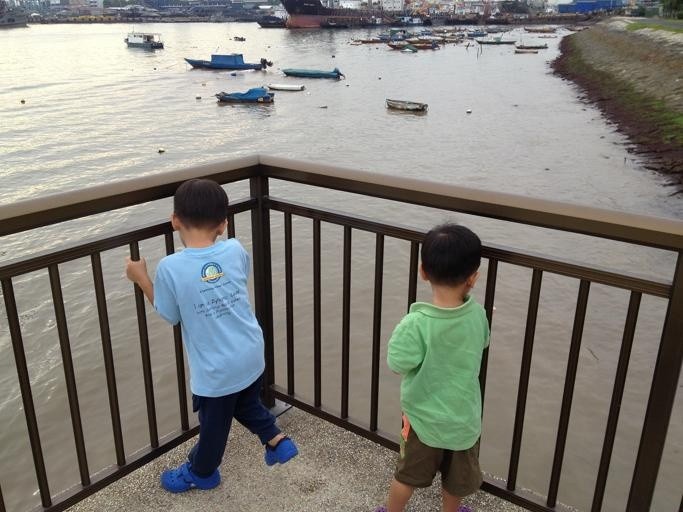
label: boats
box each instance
[384,96,428,114]
[214,84,276,104]
[565,25,589,32]
[182,53,273,71]
[280,67,343,81]
[122,31,164,49]
[265,83,306,91]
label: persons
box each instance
[123,177,297,493]
[366,222,492,511]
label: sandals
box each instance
[160,462,220,493]
[265,436,299,466]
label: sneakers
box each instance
[456,506,472,512]
[372,506,390,512]
